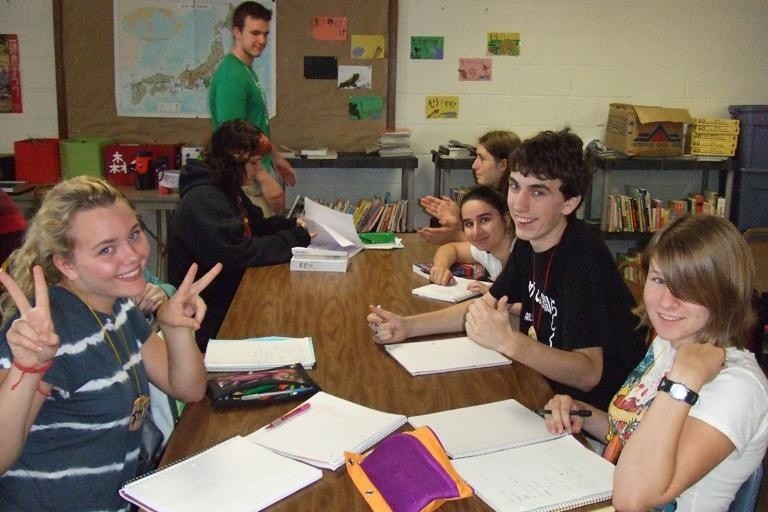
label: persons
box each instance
[429,186,522,316]
[120,264,216,443]
[544,215,767,512]
[209,1,296,219]
[170,119,316,348]
[366,128,644,452]
[1,176,223,512]
[419,131,520,245]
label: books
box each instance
[608,184,725,286]
[203,337,316,372]
[385,336,513,378]
[244,391,408,472]
[118,434,324,512]
[274,126,493,303]
[407,396,615,512]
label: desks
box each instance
[1,181,186,283]
[129,234,652,511]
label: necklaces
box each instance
[61,279,151,431]
[232,50,270,127]
[603,342,669,465]
[530,241,561,327]
[237,195,251,239]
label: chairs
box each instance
[617,225,768,510]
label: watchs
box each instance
[657,378,698,406]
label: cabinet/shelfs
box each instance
[580,148,738,244]
[430,147,482,229]
[275,149,421,234]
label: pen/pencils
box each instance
[530,410,592,416]
[217,366,314,401]
[265,403,310,430]
[374,304,382,336]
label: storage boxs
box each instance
[13,136,185,192]
[602,101,694,158]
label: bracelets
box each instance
[10,359,52,390]
